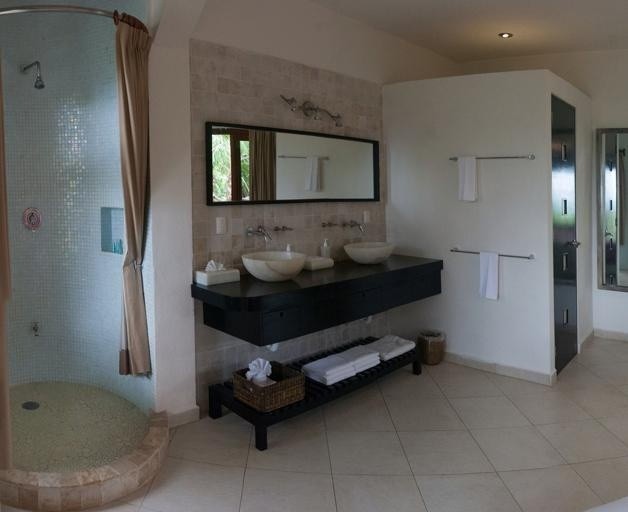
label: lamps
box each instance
[280,93,345,131]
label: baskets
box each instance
[232,360,306,413]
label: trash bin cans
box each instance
[418,330,443,365]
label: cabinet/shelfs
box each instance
[258,270,440,345]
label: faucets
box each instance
[256,224,273,241]
[352,220,367,234]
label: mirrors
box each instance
[594,127,628,292]
[204,120,380,206]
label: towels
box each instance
[477,251,500,301]
[304,155,322,193]
[301,334,417,386]
[455,156,479,202]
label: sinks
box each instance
[344,240,395,265]
[241,249,308,282]
